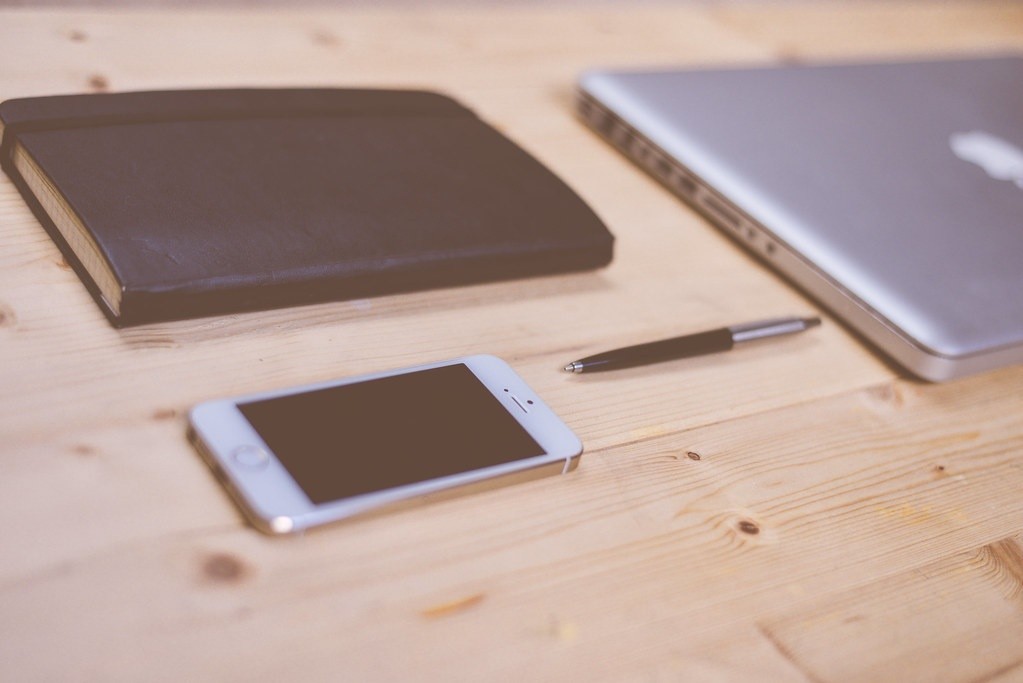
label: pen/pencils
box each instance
[564,317,823,374]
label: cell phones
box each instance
[188,352,584,536]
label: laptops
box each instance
[571,49,1022,382]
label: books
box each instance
[1,86,614,328]
